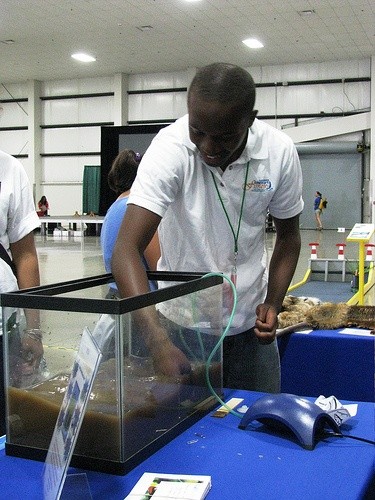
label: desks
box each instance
[39,215,105,236]
[277,327,375,403]
[0,388,375,500]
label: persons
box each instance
[110,63,304,394]
[0,149,44,439]
[38,196,48,215]
[100,149,161,300]
[313,191,328,231]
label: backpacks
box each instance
[318,196,328,210]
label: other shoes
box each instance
[315,226,323,231]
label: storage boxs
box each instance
[0,270,224,476]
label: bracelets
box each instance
[23,329,43,343]
